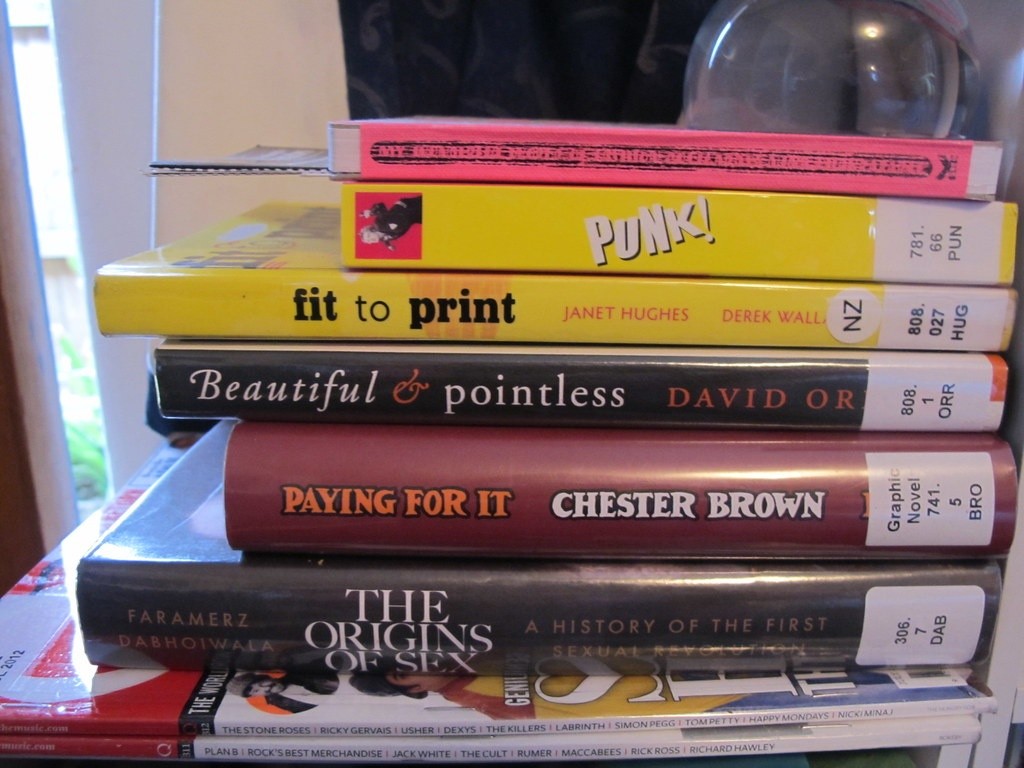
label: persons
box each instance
[226,667,339,716]
[348,669,972,716]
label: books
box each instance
[340,183,1019,288]
[75,417,1002,667]
[153,338,1009,433]
[94,199,1018,353]
[328,115,1004,203]
[1,441,1000,763]
[225,421,1019,562]
